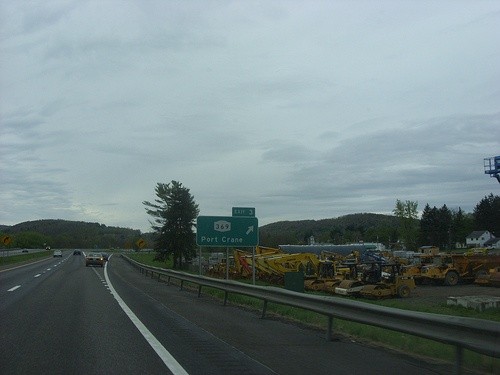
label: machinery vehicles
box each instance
[206,244,500,300]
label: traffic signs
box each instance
[196,215,260,246]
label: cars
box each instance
[53,250,62,257]
[85,252,104,267]
[73,249,82,255]
[101,252,109,261]
[45,246,51,250]
[22,248,29,253]
